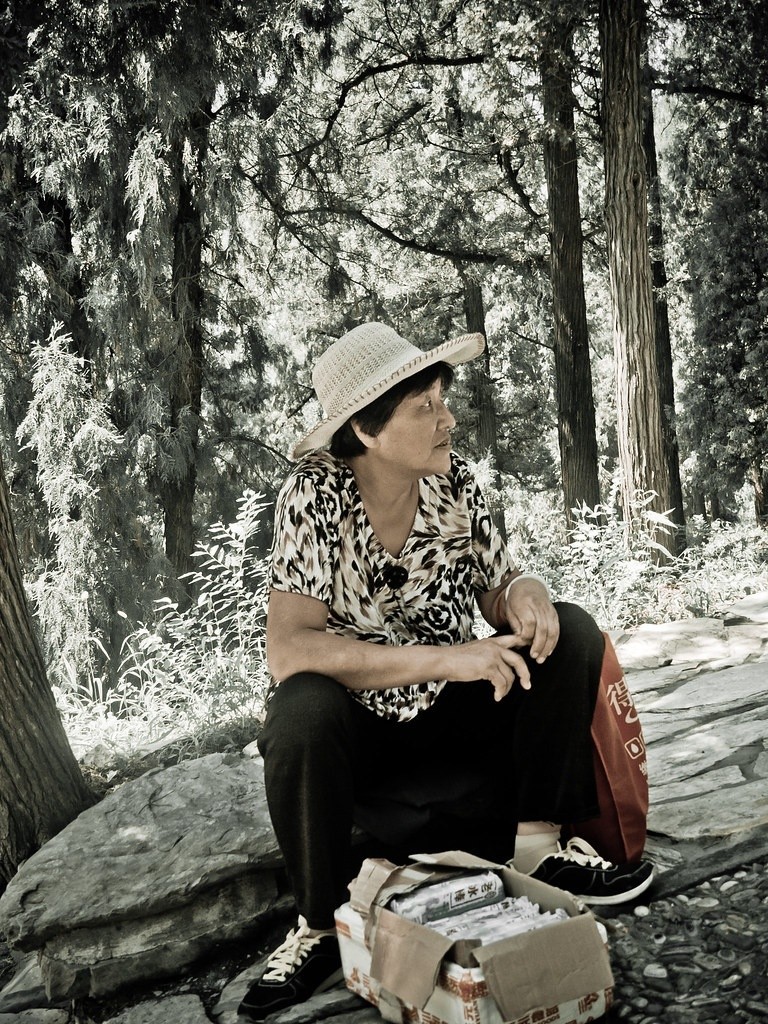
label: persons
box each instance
[238,320,658,1024]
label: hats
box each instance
[291,321,485,459]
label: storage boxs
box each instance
[330,848,615,1024]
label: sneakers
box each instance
[237,915,344,1023]
[505,838,656,906]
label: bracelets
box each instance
[505,574,549,603]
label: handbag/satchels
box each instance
[584,631,649,863]
